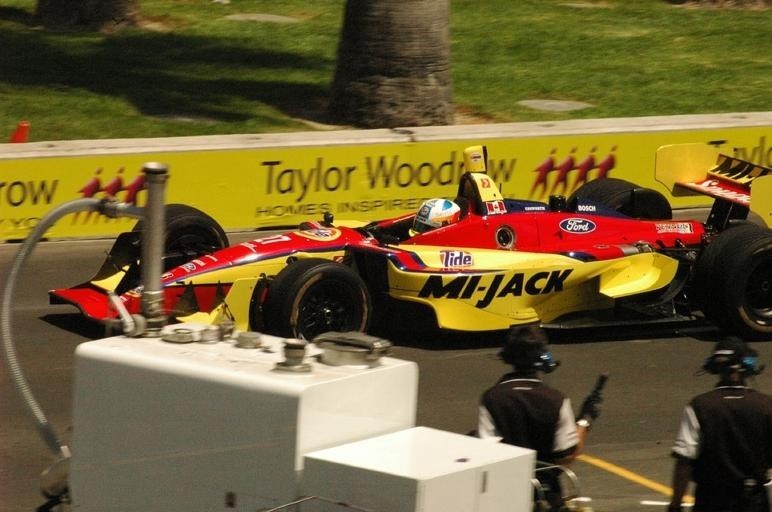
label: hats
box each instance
[713,336,759,358]
[502,325,547,365]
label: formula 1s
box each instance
[40,136,772,348]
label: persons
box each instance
[478,324,596,512]
[395,199,461,247]
[667,336,772,512]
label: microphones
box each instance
[752,364,766,375]
[545,359,561,373]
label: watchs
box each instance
[576,418,590,431]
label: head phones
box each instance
[703,336,759,373]
[499,324,554,370]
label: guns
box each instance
[576,374,608,431]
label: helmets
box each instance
[413,198,461,235]
